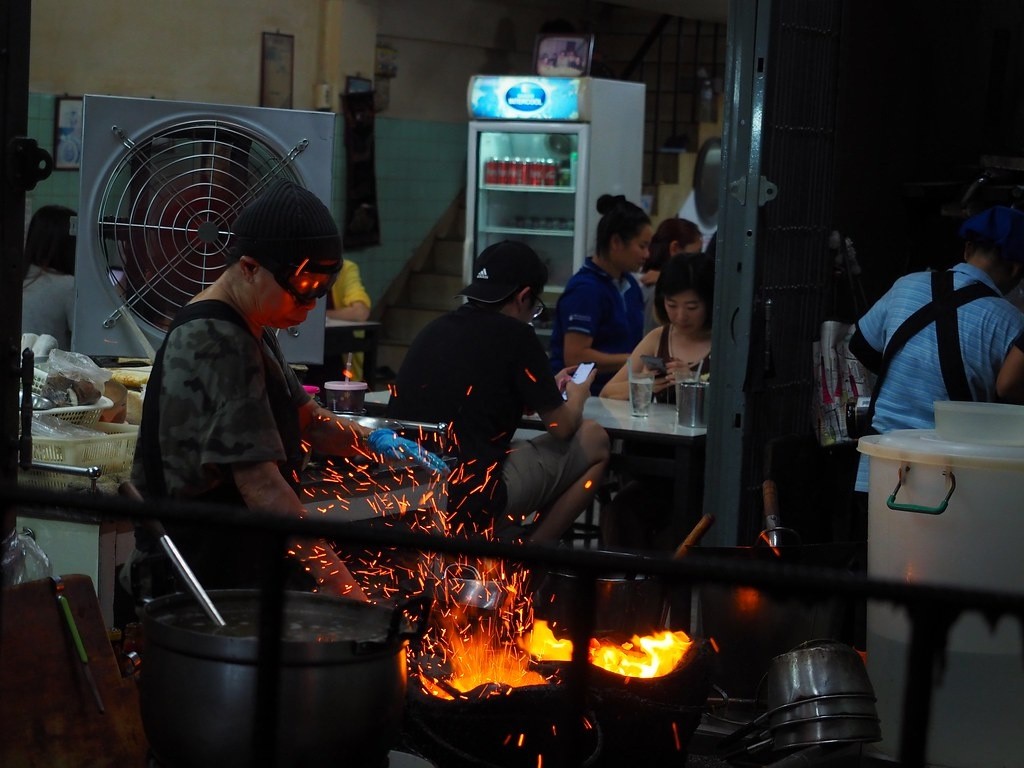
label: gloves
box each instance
[368,428,450,476]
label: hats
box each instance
[233,180,341,260]
[454,241,545,303]
[958,206,1024,255]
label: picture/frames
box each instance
[53,95,83,171]
[531,33,595,76]
[260,29,295,109]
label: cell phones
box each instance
[562,360,597,401]
[641,354,668,377]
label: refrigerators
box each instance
[466,74,646,359]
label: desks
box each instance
[364,388,706,553]
[326,320,382,379]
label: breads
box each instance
[110,370,147,387]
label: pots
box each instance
[534,550,661,630]
[707,643,883,762]
[128,556,436,768]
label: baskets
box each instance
[20,364,115,428]
[19,419,141,476]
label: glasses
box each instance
[275,264,338,304]
[534,296,545,318]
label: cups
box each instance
[676,371,701,412]
[325,381,368,416]
[679,381,709,431]
[627,357,656,419]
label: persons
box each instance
[125,178,453,606]
[382,236,612,549]
[848,205,1024,493]
[21,205,126,349]
[309,258,373,403]
[599,250,715,551]
[547,194,655,397]
[637,216,717,339]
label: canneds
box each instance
[486,156,560,186]
[570,151,578,188]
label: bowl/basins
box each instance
[933,402,1024,446]
[100,381,128,424]
[19,389,56,409]
